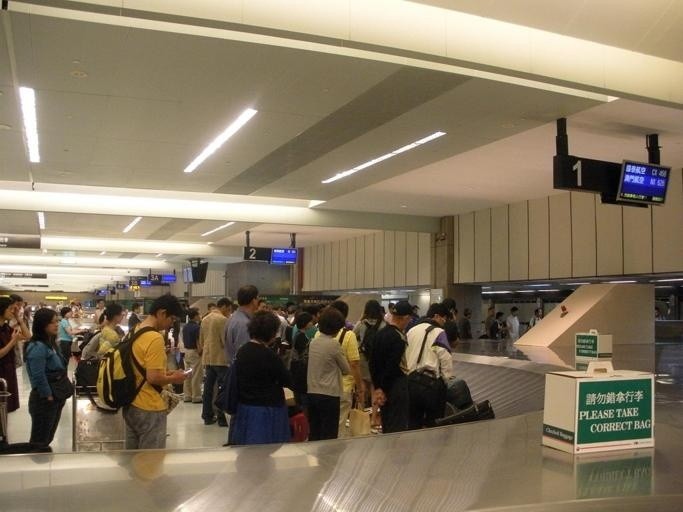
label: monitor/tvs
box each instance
[138,273,176,285]
[601,194,648,208]
[270,248,298,265]
[183,262,208,283]
[616,159,672,205]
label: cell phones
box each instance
[184,368,192,374]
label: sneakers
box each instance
[204,417,228,427]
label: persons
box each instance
[182,285,461,450]
[81,294,186,450]
[1,294,88,449]
[655,306,666,320]
[458,307,474,354]
[530,308,543,328]
[484,306,520,356]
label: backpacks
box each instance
[96,324,164,410]
[358,318,383,361]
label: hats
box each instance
[392,300,418,318]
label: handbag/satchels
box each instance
[213,358,239,415]
[70,342,81,353]
[13,343,23,369]
[407,367,495,425]
[76,359,101,393]
[350,408,373,437]
[48,374,74,401]
